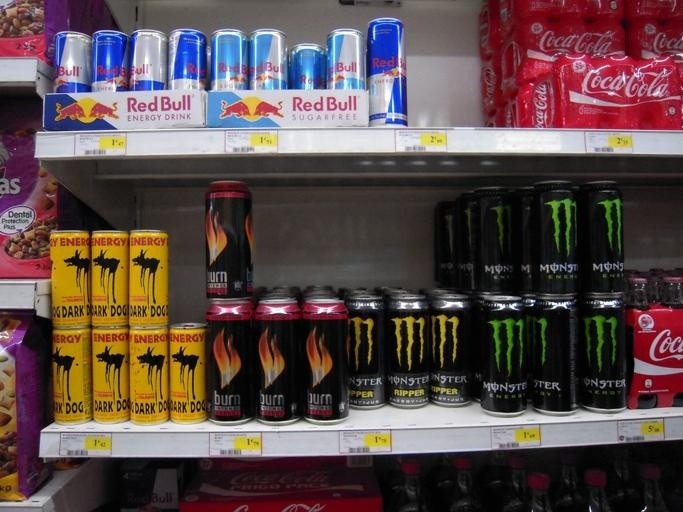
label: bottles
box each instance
[378,444,683,512]
[623,264,683,410]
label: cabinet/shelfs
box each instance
[34,127,683,458]
[0,55,111,512]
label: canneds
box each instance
[204,178,630,425]
[48,229,208,422]
[478,0,683,130]
[52,16,408,127]
